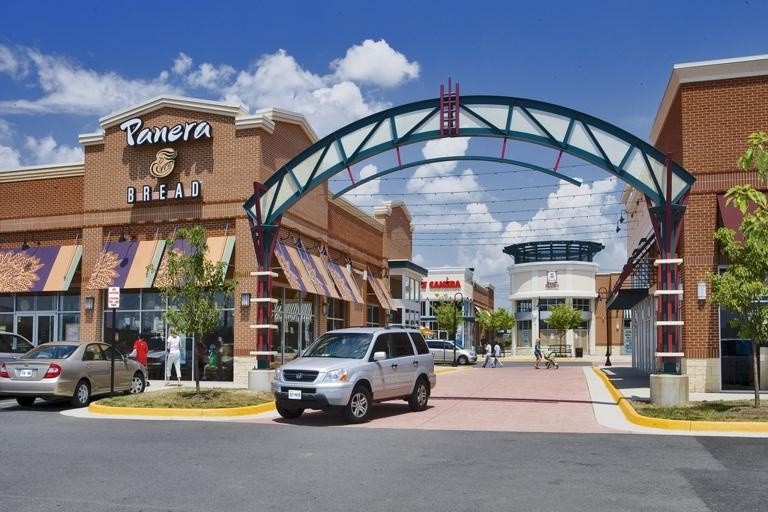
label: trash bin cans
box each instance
[575,348,583,358]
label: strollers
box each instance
[542,350,559,370]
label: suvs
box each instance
[423,338,477,366]
[269,326,436,422]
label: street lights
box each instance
[452,291,465,365]
[596,287,611,365]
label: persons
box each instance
[480,342,496,368]
[492,341,503,368]
[533,338,543,369]
[163,326,182,386]
[127,334,150,387]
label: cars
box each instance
[130,334,195,378]
[1,340,149,407]
[0,331,35,363]
